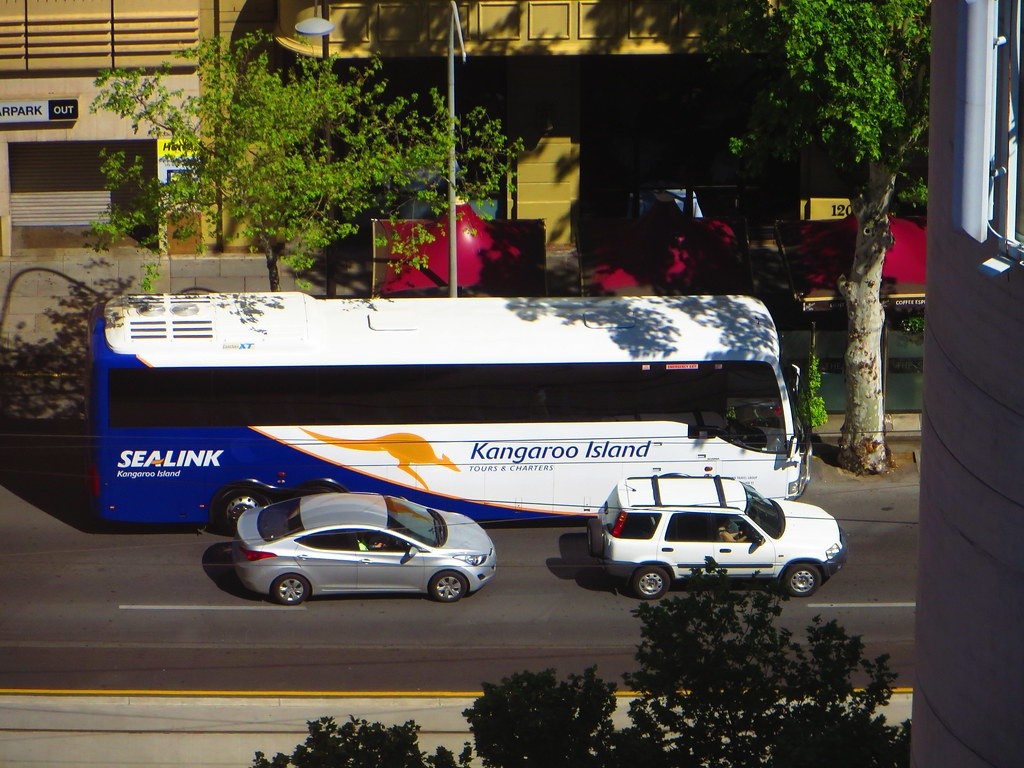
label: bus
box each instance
[88,291,816,539]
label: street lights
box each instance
[443,0,469,299]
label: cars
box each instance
[233,492,498,603]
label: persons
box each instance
[716,514,753,543]
[363,531,404,552]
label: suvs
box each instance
[586,474,849,601]
